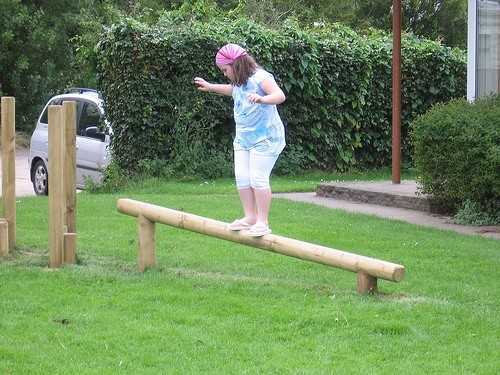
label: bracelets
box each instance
[209,83,213,94]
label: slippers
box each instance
[226,215,272,238]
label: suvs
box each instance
[28,87,115,197]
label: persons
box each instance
[194,44,286,237]
